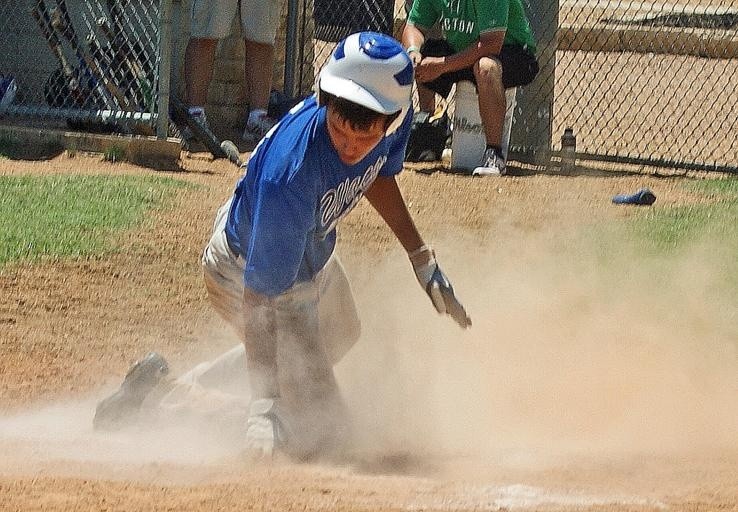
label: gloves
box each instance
[244,397,297,464]
[407,243,472,329]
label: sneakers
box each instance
[242,112,277,143]
[190,111,220,146]
[91,351,169,431]
[413,108,433,129]
[470,142,507,177]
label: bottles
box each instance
[560,128,576,175]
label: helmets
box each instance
[315,31,415,139]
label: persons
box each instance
[400,0,541,177]
[95,28,471,475]
[180,1,287,155]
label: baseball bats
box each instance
[32,0,229,159]
